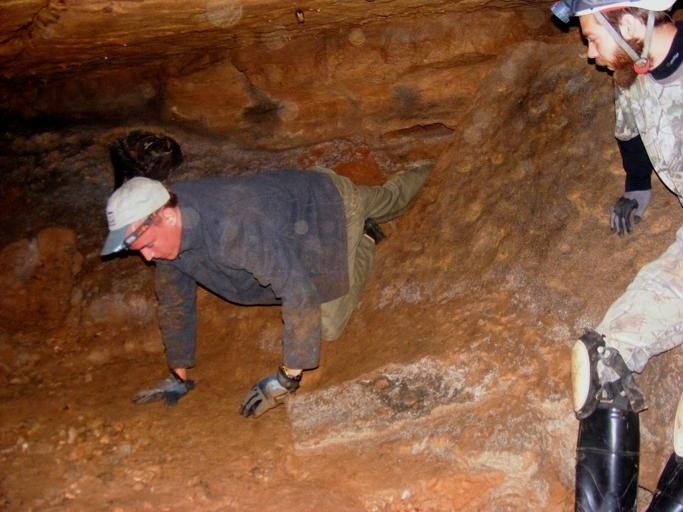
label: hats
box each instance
[100,177,171,257]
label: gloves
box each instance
[237,366,301,418]
[609,190,652,239]
[133,366,192,406]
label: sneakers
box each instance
[363,218,384,243]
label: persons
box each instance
[96,165,435,419]
[548,1,682,510]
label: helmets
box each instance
[575,0,675,18]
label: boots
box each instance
[574,409,682,511]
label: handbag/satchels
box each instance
[110,132,183,182]
